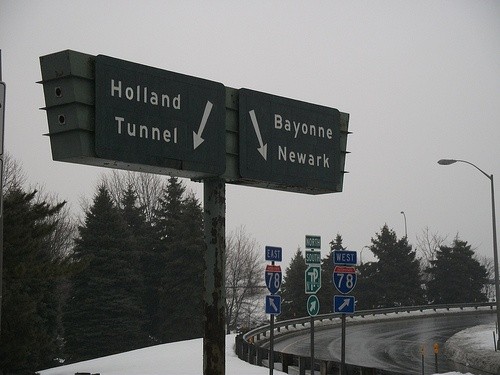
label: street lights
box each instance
[400,211,408,238]
[437,158,500,346]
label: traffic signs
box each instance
[236,87,344,189]
[92,54,226,176]
